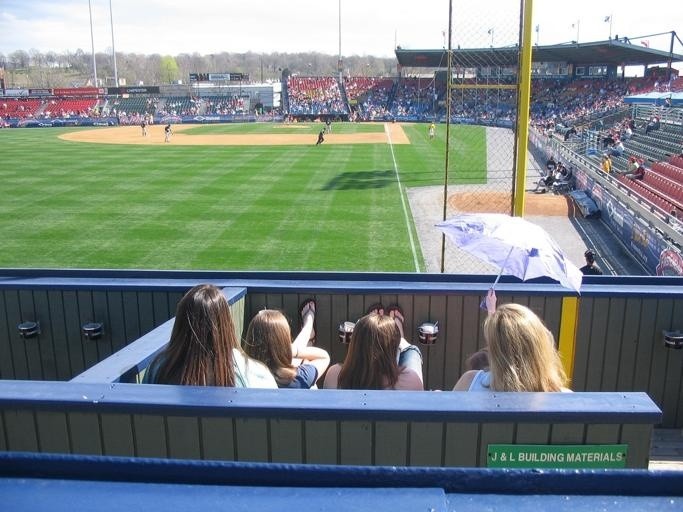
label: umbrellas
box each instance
[434,212,585,312]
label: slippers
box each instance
[387,306,404,328]
[300,299,317,341]
[369,304,384,317]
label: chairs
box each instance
[610,123,683,222]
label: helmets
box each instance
[585,249,596,257]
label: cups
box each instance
[342,321,356,331]
[421,322,436,333]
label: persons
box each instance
[578,249,604,275]
[323,303,424,391]
[141,123,147,136]
[1,92,273,124]
[450,289,572,391]
[531,68,679,191]
[275,70,518,122]
[241,297,331,389]
[140,283,277,390]
[429,122,435,139]
[164,124,174,142]
[316,122,332,146]
